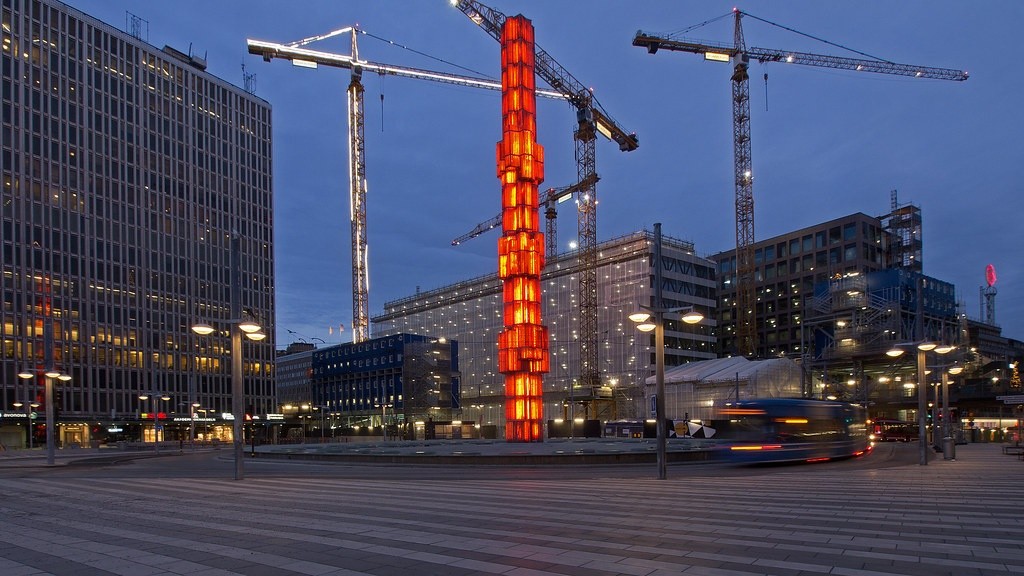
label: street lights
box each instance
[284,402,309,443]
[191,313,267,482]
[198,408,216,445]
[14,400,42,449]
[374,401,393,442]
[888,337,950,466]
[329,411,341,437]
[177,400,200,444]
[627,301,704,478]
[313,405,331,437]
[17,367,72,464]
[562,399,583,439]
[923,362,964,448]
[140,391,171,455]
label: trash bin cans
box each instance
[942,436,955,460]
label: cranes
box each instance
[453,1,640,396]
[245,24,581,343]
[454,174,603,259]
[631,7,970,361]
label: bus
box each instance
[713,396,873,468]
[874,419,920,442]
